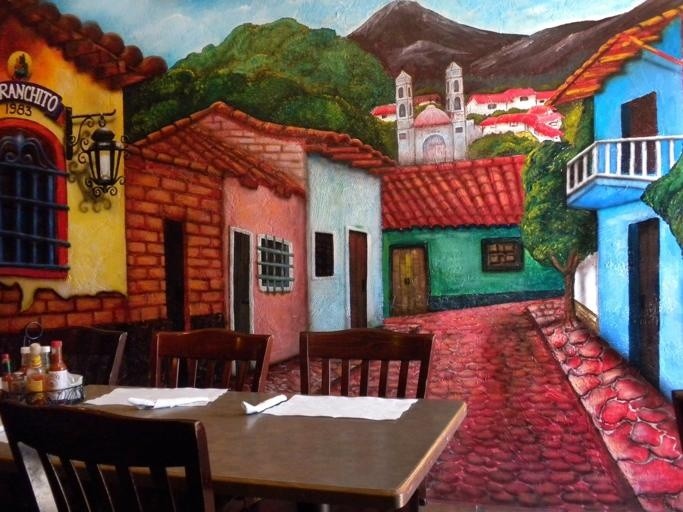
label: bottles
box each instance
[3,337,68,405]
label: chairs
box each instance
[18,326,128,386]
[153,328,273,392]
[299,328,434,398]
[0,400,216,511]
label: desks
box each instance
[0,371,468,510]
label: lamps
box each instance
[64,104,131,198]
